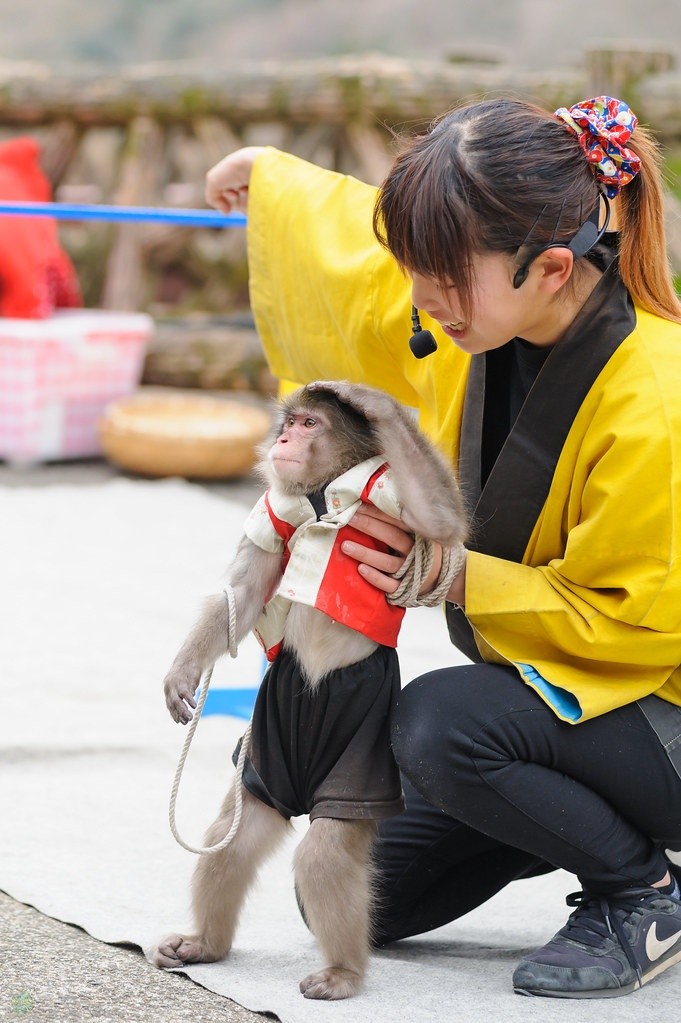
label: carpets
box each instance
[3,472,680,1023]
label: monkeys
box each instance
[154,380,467,1002]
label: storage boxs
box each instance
[97,388,268,479]
[0,304,155,468]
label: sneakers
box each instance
[513,863,681,1000]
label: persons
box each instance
[206,83,681,1002]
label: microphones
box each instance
[409,305,438,359]
